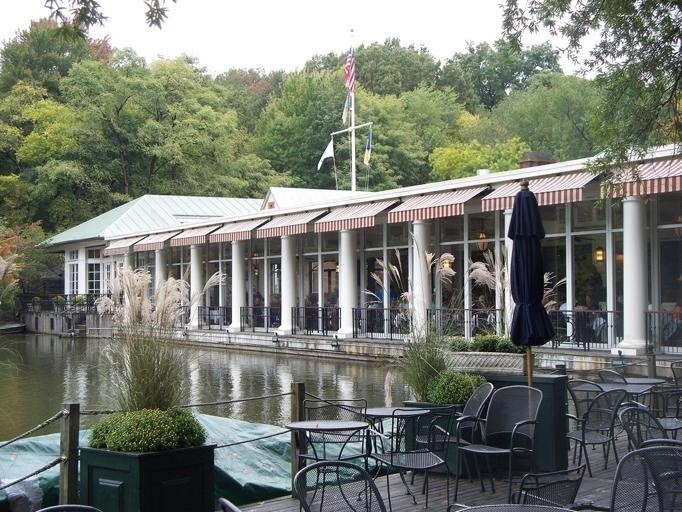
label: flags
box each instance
[341,46,355,124]
[315,140,333,171]
[364,129,373,167]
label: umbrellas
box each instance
[507,178,555,472]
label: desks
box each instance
[359,406,428,504]
[576,378,653,443]
[616,374,666,422]
[643,416,681,442]
[284,420,369,511]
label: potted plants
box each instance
[77,262,228,512]
[357,230,497,477]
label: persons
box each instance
[661,304,682,346]
[430,288,463,335]
[357,298,408,333]
[255,290,263,306]
[541,292,623,343]
[472,295,495,327]
[329,298,339,330]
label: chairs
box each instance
[671,361,681,420]
[618,405,681,499]
[566,376,630,461]
[565,388,623,482]
[454,384,544,505]
[595,367,650,441]
[604,438,682,511]
[370,406,453,512]
[510,463,586,511]
[297,399,369,510]
[413,382,491,500]
